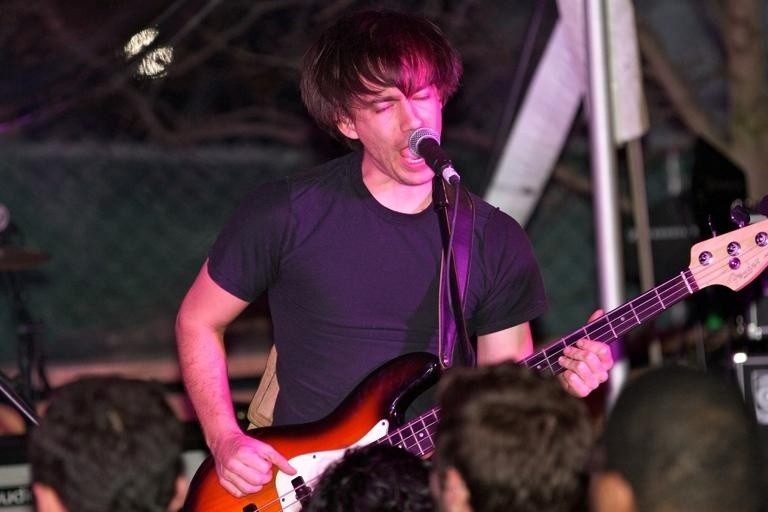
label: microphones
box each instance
[408,127,461,186]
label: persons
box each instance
[588,365,768,512]
[174,11,613,499]
[302,443,441,512]
[31,373,187,512]
[430,357,596,511]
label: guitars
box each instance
[184,199,768,512]
